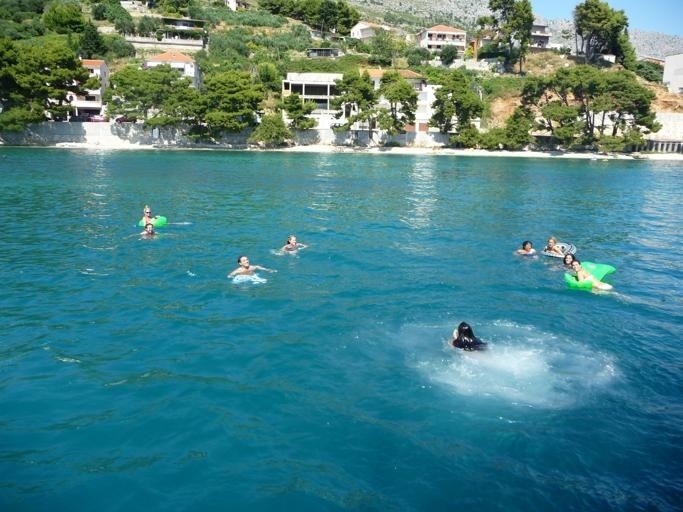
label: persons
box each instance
[516,237,613,291]
[452,321,484,351]
[230,255,273,279]
[281,235,304,252]
[140,224,155,237]
[143,205,160,224]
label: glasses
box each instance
[147,212,151,214]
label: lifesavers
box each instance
[139,216,167,227]
[541,242,577,260]
